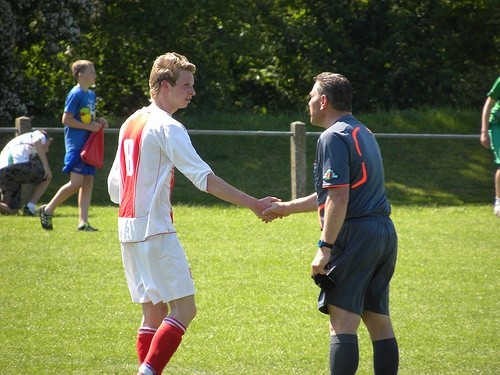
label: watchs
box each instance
[318,240,333,249]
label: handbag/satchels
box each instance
[80,123,105,169]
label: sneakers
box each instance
[78,223,98,231]
[39,204,53,230]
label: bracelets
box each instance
[481,131,486,133]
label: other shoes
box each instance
[22,207,39,216]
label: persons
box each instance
[261,72,399,375]
[108,54,281,374]
[39,60,108,232]
[0,130,52,216]
[480,77,500,217]
